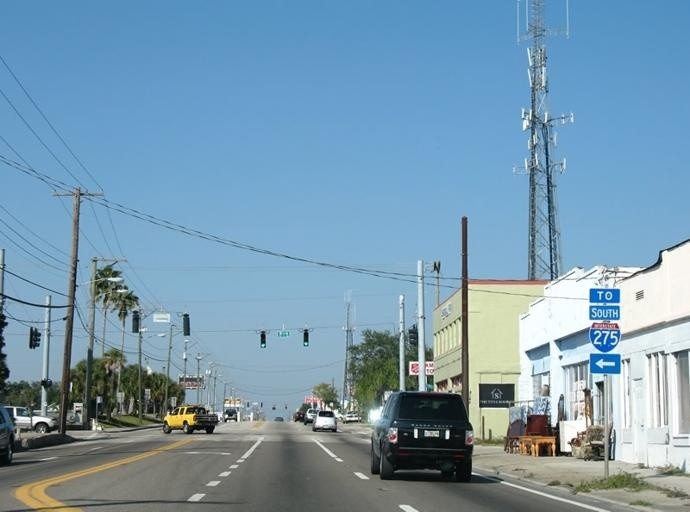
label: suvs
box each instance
[313,410,337,432]
[369,390,474,481]
[4,405,57,434]
[224,409,237,422]
[304,408,320,425]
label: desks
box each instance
[519,435,556,457]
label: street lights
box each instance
[58,276,124,437]
[138,329,166,426]
[84,287,130,426]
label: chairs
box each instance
[504,419,524,454]
[571,422,613,461]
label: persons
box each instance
[539,383,549,396]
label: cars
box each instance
[275,416,284,422]
[342,412,362,424]
[293,411,305,422]
[0,405,15,463]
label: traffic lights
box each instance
[261,334,266,348]
[303,332,308,346]
[40,380,47,386]
[33,328,41,349]
[29,327,37,348]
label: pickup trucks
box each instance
[163,405,218,433]
